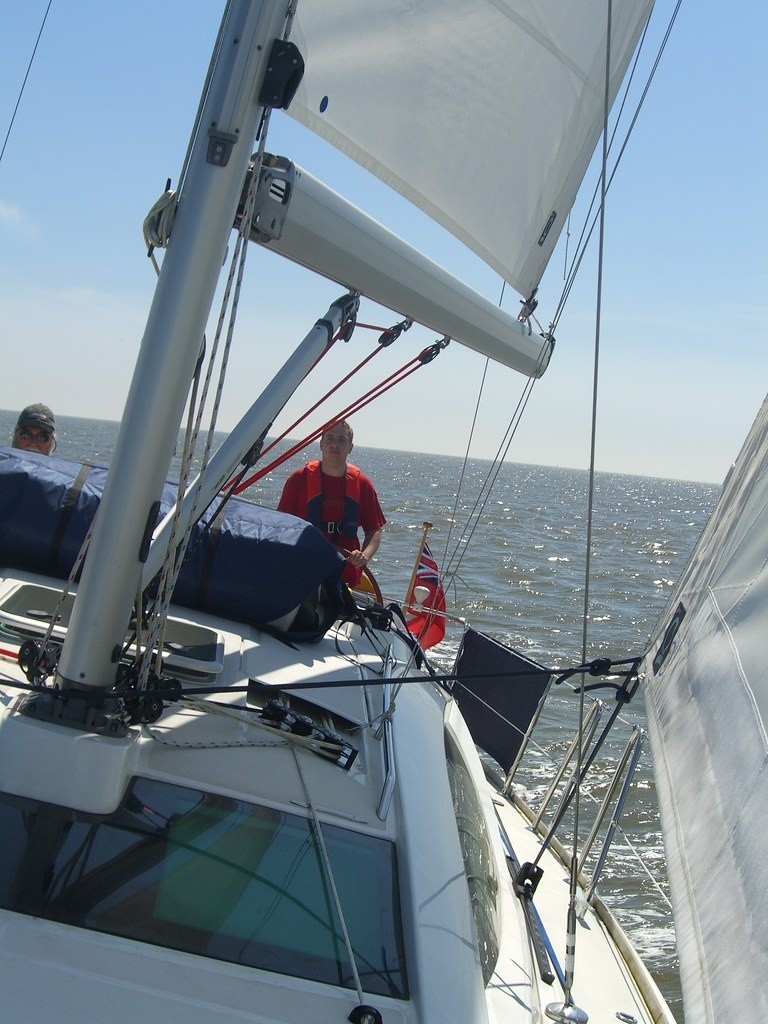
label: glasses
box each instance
[19,430,51,442]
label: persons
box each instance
[11,402,56,456]
[276,420,387,589]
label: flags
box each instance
[401,541,446,650]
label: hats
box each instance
[18,404,55,435]
